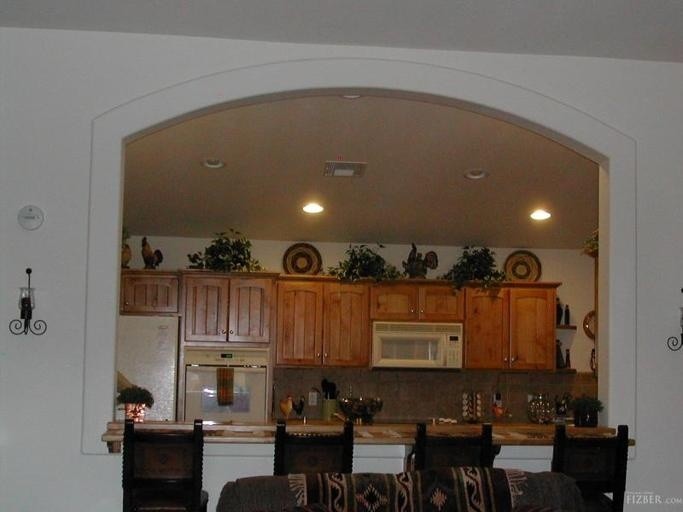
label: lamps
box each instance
[7,267,46,336]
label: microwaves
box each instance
[368,320,465,371]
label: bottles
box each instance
[556,296,573,370]
[526,390,555,424]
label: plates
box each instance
[583,309,595,340]
[504,250,542,282]
[281,242,323,276]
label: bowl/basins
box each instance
[340,397,382,426]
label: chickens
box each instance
[292,395,306,419]
[141,237,163,269]
[279,395,293,420]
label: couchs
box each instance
[218,467,587,512]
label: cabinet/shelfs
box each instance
[176,271,279,352]
[464,278,564,375]
[556,322,578,376]
[273,273,371,367]
[118,271,181,317]
[366,277,466,322]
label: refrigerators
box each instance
[114,314,180,422]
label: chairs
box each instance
[546,425,630,512]
[271,421,356,475]
[118,417,211,512]
[402,421,502,478]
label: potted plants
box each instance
[570,395,603,427]
[114,387,155,423]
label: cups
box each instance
[320,398,337,422]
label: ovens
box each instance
[180,346,271,424]
